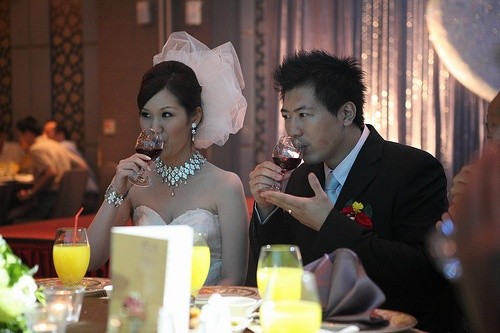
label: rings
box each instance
[288,209,292,213]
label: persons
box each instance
[250,46,460,333]
[81,31,249,285]
[1,117,90,225]
[43,121,100,215]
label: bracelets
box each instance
[103,184,130,208]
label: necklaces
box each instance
[154,151,207,197]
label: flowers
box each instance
[342,200,375,232]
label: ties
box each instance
[325,174,340,206]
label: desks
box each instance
[0,214,136,277]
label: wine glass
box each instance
[263,134,305,191]
[127,130,165,188]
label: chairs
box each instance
[19,168,93,221]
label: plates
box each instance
[34,277,112,296]
[249,308,417,333]
[200,285,260,300]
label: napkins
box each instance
[304,248,389,331]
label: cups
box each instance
[53,226,91,286]
[203,295,258,333]
[24,283,85,333]
[257,244,322,332]
[104,286,112,297]
[191,233,211,296]
